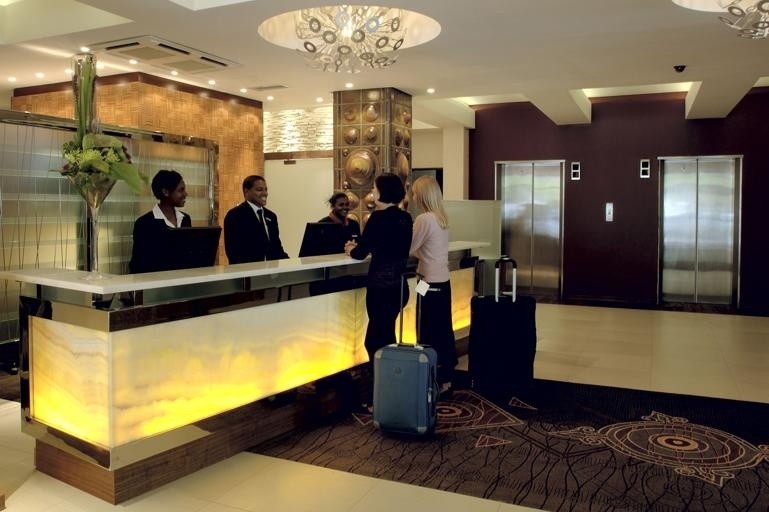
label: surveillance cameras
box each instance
[673,65,686,73]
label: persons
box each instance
[318,193,363,248]
[127,171,192,272]
[224,176,290,264]
[344,172,413,413]
[407,175,457,399]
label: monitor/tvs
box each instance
[298,223,344,258]
[155,226,222,272]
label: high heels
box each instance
[447,386,452,397]
[440,389,448,403]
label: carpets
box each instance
[244,369,769,511]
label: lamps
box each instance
[295,4,407,75]
[717,0,769,40]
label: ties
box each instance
[257,209,267,241]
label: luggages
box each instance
[468,256,538,392]
[372,273,442,437]
